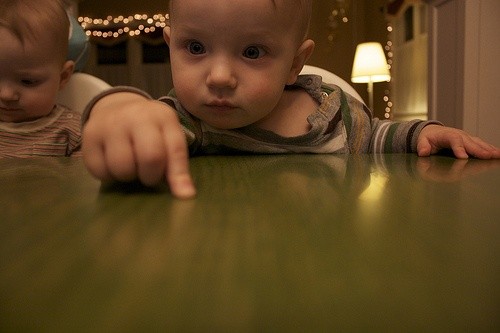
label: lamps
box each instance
[351,42,389,118]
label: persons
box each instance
[1,0,84,157]
[61,2,90,63]
[81,0,500,198]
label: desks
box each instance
[0,151,500,333]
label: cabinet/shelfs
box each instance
[386,0,432,122]
